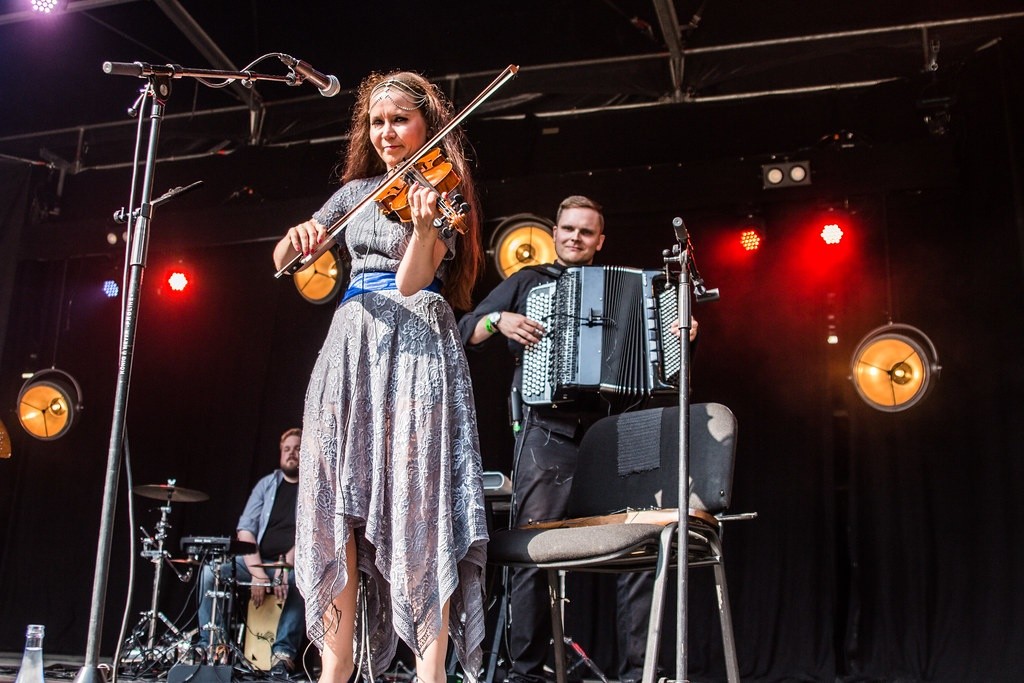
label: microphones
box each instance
[541,317,547,321]
[278,52,341,97]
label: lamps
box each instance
[849,204,941,413]
[16,260,86,442]
[293,246,343,305]
[761,161,812,190]
[486,212,558,280]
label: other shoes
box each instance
[346,664,364,683]
[271,653,295,674]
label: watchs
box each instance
[490,308,503,332]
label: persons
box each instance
[272,71,490,683]
[198,428,303,681]
[460,195,699,683]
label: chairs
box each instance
[486,403,741,683]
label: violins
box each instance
[373,146,471,240]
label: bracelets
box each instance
[485,314,495,334]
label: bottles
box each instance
[12,624,46,683]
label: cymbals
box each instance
[169,559,205,566]
[250,561,294,568]
[132,484,208,502]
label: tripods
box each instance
[107,492,262,680]
[539,569,609,683]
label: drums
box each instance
[244,595,285,671]
